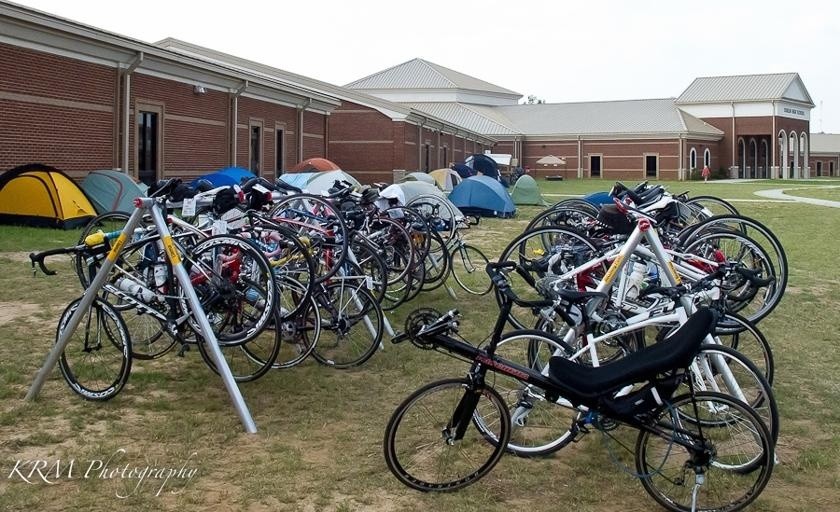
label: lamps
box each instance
[193,85,207,94]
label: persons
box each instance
[701,165,711,185]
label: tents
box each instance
[0,163,106,230]
[378,154,551,231]
[79,169,152,221]
[184,165,259,191]
[274,157,363,197]
